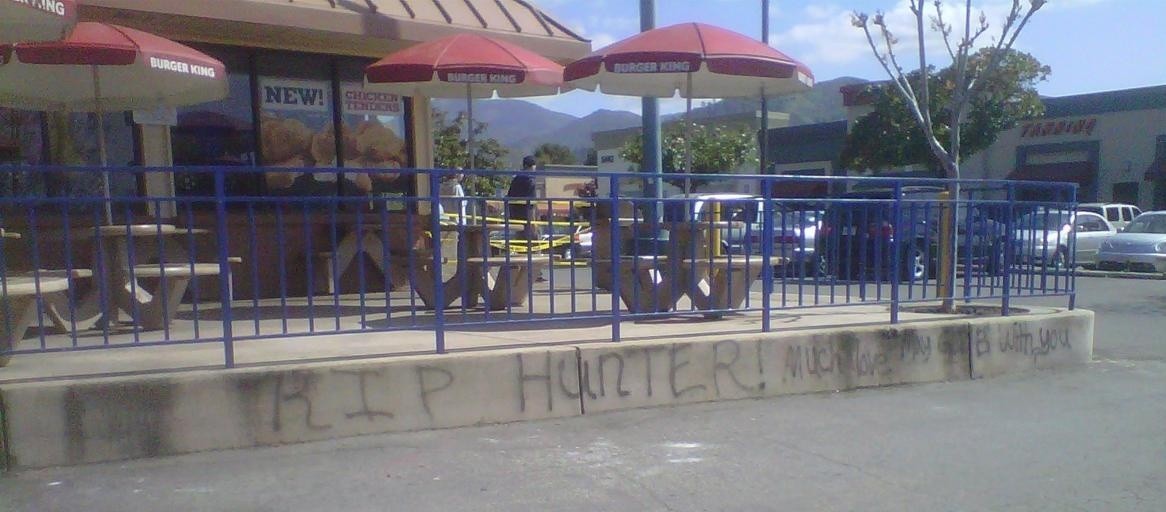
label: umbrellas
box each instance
[0,0,79,44]
[0,20,231,227]
[362,33,563,224]
[563,21,813,223]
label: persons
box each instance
[438,166,467,224]
[507,156,537,220]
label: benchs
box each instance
[0,257,241,368]
[587,255,790,313]
[312,249,561,311]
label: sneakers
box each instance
[535,277,546,281]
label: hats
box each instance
[523,156,536,168]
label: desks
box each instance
[71,224,174,330]
[629,220,744,317]
[422,224,525,308]
